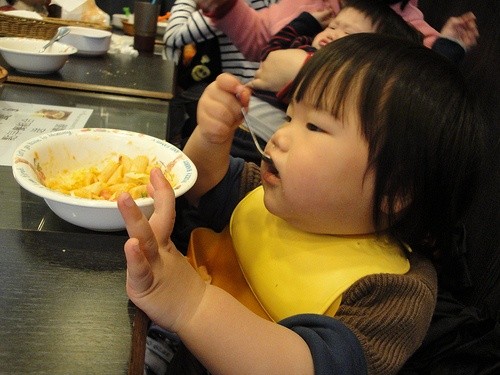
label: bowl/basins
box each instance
[0,36,78,74]
[57,26,112,56]
[11,126,198,232]
[112,14,134,35]
[154,21,167,35]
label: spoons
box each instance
[242,106,279,178]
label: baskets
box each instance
[0,12,112,41]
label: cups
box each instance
[134,1,159,53]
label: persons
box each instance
[0,0,66,33]
[116,0,500,375]
[171,0,413,171]
[119,31,493,375]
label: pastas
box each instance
[48,156,166,202]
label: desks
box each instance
[0,27,178,375]
